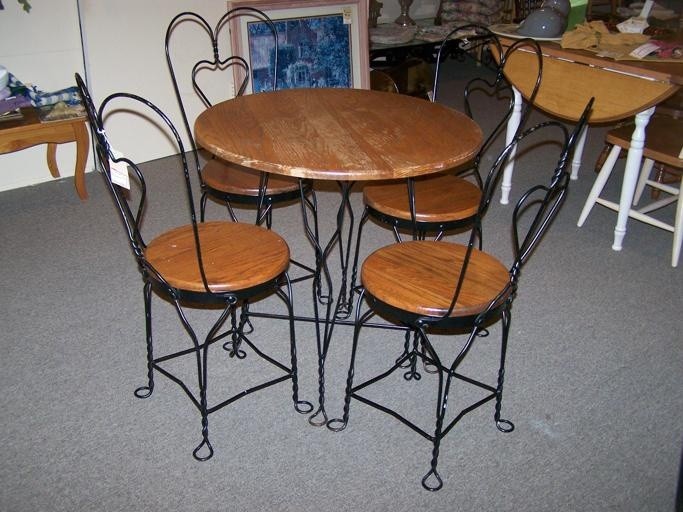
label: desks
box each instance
[0,106,91,201]
[487,22,683,204]
[369,20,496,69]
[194,87,484,320]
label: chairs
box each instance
[577,118,683,268]
[75,73,313,461]
[336,23,543,316]
[166,6,333,305]
[327,96,595,491]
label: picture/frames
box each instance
[227,0,370,96]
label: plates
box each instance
[488,23,563,41]
